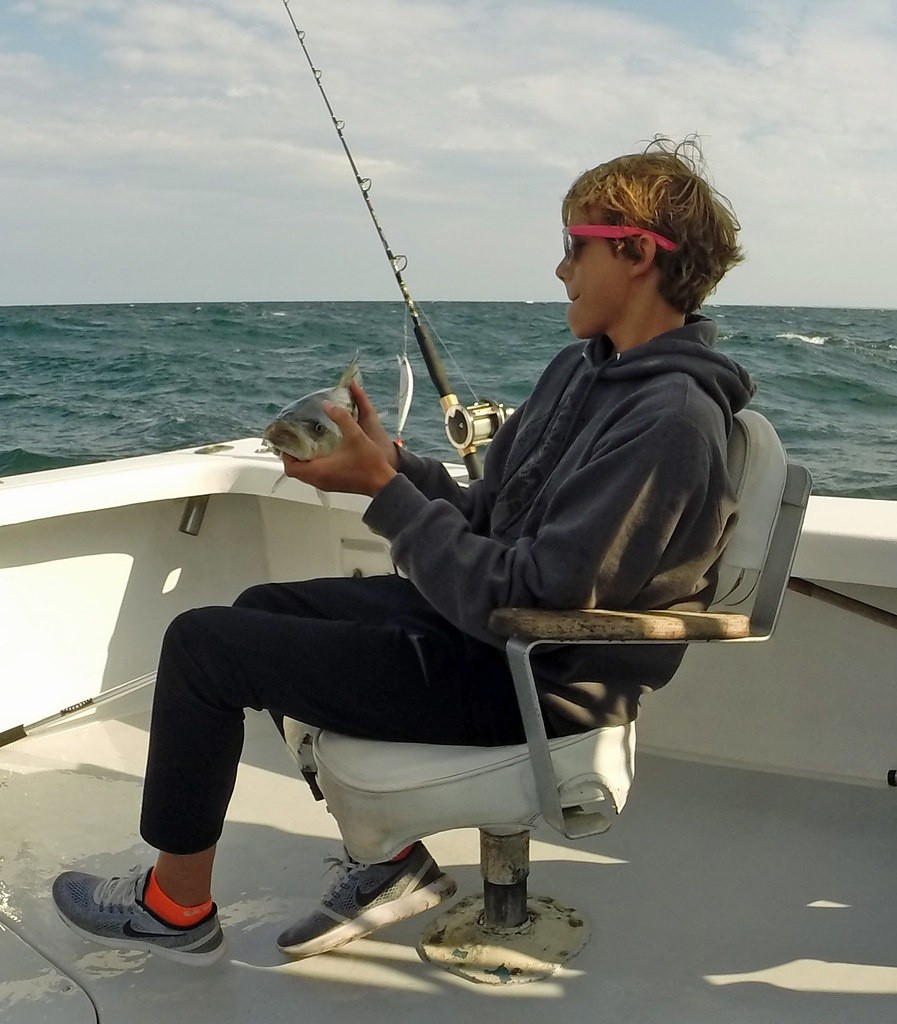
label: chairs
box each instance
[310,408,819,990]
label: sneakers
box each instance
[276,840,459,959]
[51,864,226,969]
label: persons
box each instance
[50,133,759,968]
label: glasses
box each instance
[561,224,680,259]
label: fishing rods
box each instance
[282,0,503,481]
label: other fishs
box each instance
[261,356,366,509]
